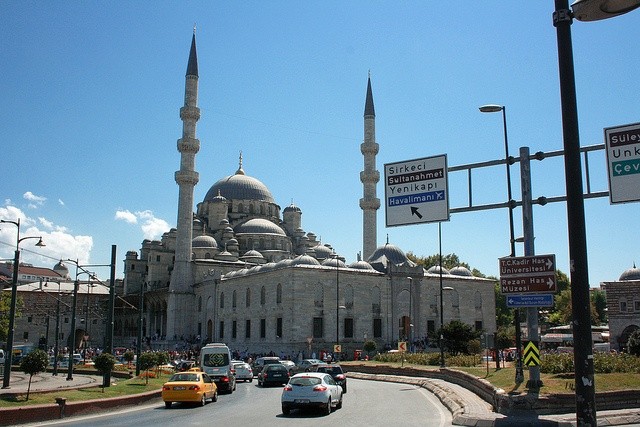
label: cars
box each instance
[299,359,327,372]
[162,371,218,408]
[282,371,343,413]
[233,363,253,382]
[258,363,290,387]
[205,367,236,393]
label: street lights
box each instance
[54,258,97,381]
[407,276,412,348]
[553,0,640,427]
[441,287,453,368]
[44,280,60,375]
[337,306,345,360]
[330,254,339,306]
[0,220,46,389]
[479,105,523,382]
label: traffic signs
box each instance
[384,153,450,227]
[500,253,556,275]
[500,275,557,293]
[603,122,640,204]
[507,294,553,306]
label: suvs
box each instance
[316,365,346,392]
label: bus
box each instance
[201,342,231,371]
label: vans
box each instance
[0,349,5,363]
[73,354,82,361]
